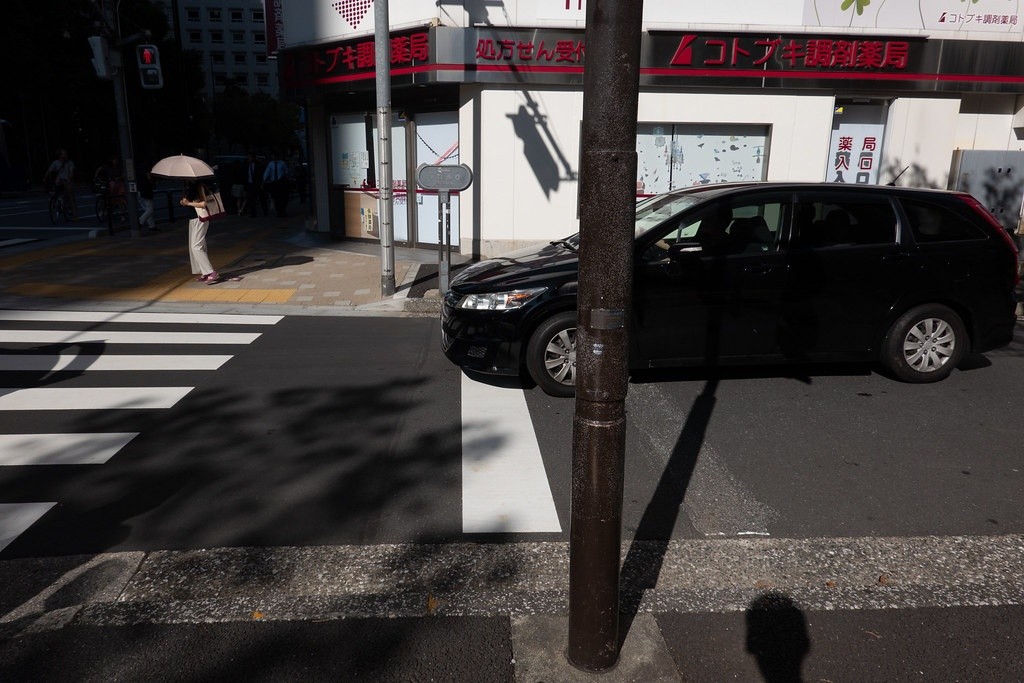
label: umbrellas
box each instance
[151,153,214,177]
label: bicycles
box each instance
[47,182,73,225]
[94,177,128,224]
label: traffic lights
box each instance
[137,46,163,89]
[87,37,112,81]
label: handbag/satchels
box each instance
[271,181,278,198]
[193,184,226,221]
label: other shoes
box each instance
[263,210,268,216]
[205,273,220,284]
[196,275,208,281]
[137,217,143,227]
[275,213,288,217]
[150,227,160,231]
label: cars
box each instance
[206,155,266,209]
[438,180,1021,398]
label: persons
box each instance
[166,178,177,223]
[263,148,308,217]
[138,176,160,231]
[42,146,79,223]
[95,151,129,221]
[180,177,220,284]
[231,151,269,218]
